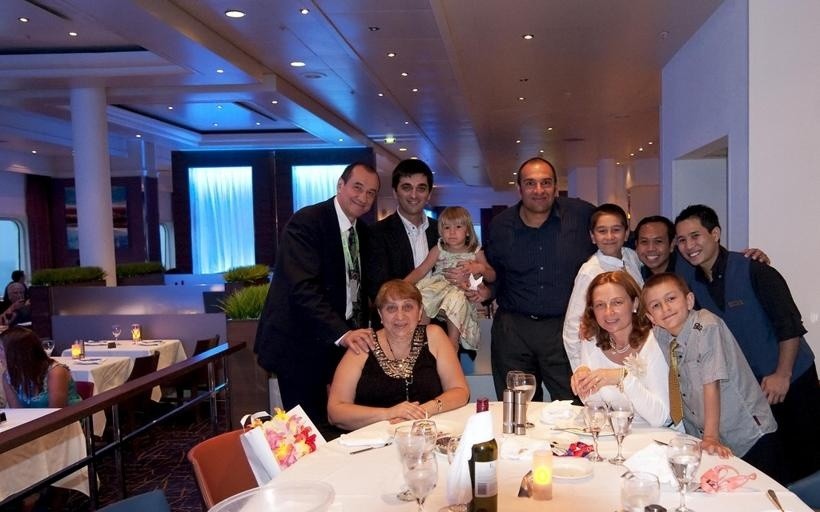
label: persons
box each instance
[462,157,636,402]
[562,204,647,374]
[637,272,780,488]
[13,271,29,292]
[0,283,34,328]
[571,269,688,434]
[359,160,478,335]
[405,206,499,350]
[254,162,379,438]
[634,215,769,291]
[0,328,85,431]
[326,276,470,440]
[674,205,819,486]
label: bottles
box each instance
[502,387,528,436]
[465,397,500,512]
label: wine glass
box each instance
[667,437,702,511]
[111,325,123,346]
[584,399,636,468]
[394,419,464,512]
[507,369,538,428]
[42,338,57,357]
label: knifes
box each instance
[349,436,393,456]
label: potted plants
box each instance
[223,263,270,298]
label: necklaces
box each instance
[608,335,632,356]
[384,333,413,361]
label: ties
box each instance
[667,336,685,428]
[345,226,361,308]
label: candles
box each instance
[531,443,556,505]
[132,325,141,343]
[72,343,81,359]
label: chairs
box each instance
[188,430,290,508]
[172,336,224,411]
[74,380,94,401]
[98,490,179,512]
[103,351,160,459]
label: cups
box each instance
[620,471,662,511]
[130,323,143,345]
[531,455,553,501]
[70,343,82,360]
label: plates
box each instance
[560,424,616,435]
[536,456,596,481]
[137,338,163,348]
[74,357,107,364]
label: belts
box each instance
[500,305,562,323]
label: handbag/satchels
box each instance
[234,402,333,492]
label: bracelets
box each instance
[433,396,444,416]
[617,367,627,394]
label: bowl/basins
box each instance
[206,478,335,511]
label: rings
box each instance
[454,279,458,283]
[594,377,601,382]
